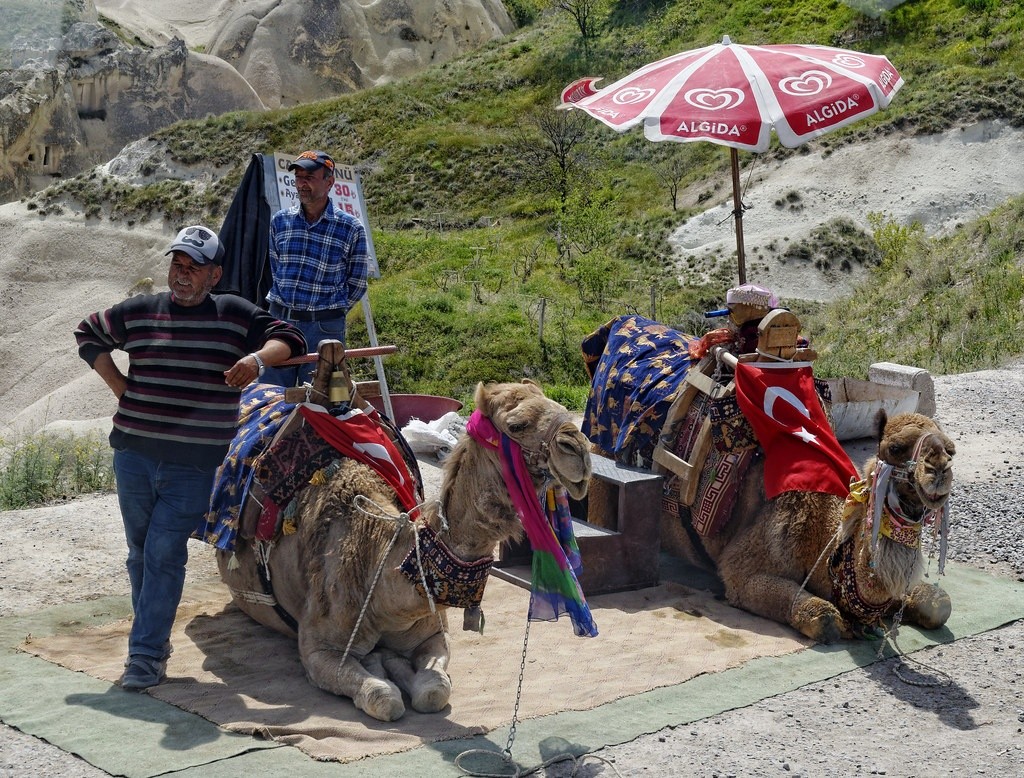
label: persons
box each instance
[264,150,367,387]
[72,225,309,689]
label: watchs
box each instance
[249,353,264,378]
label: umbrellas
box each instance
[555,33,905,287]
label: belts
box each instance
[270,301,345,322]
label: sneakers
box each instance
[123,638,170,687]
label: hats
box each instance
[165,226,225,264]
[289,149,335,173]
[727,283,772,306]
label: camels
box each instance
[563,315,952,647]
[217,379,595,722]
